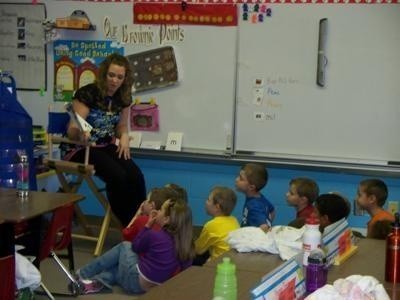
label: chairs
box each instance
[42,107,125,255]
[0,255,55,300]
[18,204,79,296]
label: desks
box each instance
[0,188,85,279]
[131,239,400,300]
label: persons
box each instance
[69,184,195,295]
[235,163,276,231]
[288,194,366,238]
[356,179,396,238]
[285,177,321,224]
[191,187,240,266]
[59,53,146,227]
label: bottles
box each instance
[302,212,321,271]
[16,155,30,201]
[386,213,399,283]
[305,250,327,291]
[213,256,238,300]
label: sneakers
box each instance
[72,268,105,294]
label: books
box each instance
[65,103,93,137]
[116,131,182,151]
